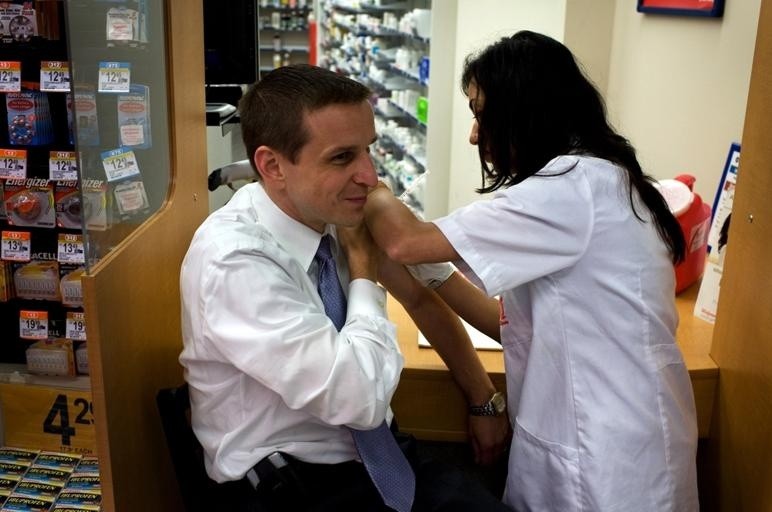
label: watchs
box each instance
[467,389,506,417]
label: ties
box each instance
[314,233,417,512]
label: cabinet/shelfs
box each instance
[259,2,567,218]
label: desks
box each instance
[387,273,715,437]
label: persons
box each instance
[361,29,699,512]
[180,65,511,512]
